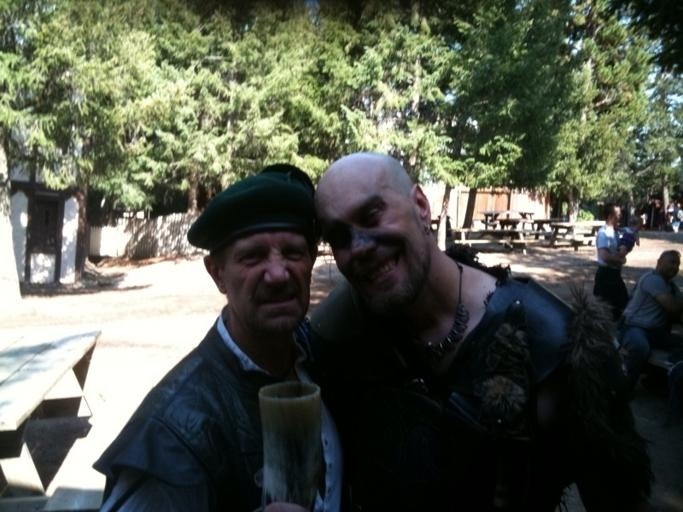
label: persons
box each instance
[310,152,656,512]
[92,162,351,512]
[618,250,683,388]
[614,216,642,257]
[593,205,629,321]
[637,196,683,234]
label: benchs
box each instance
[0,325,102,496]
[452,218,606,256]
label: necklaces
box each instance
[412,258,469,361]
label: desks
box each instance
[480,211,534,237]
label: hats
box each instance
[188,164,315,249]
